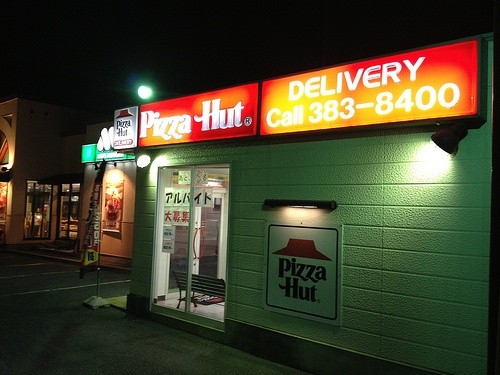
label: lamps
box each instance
[1,167,10,171]
[114,162,117,167]
[430,121,468,155]
[94,162,100,169]
[135,151,151,169]
[137,85,154,104]
[262,198,339,211]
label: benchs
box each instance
[172,270,226,310]
